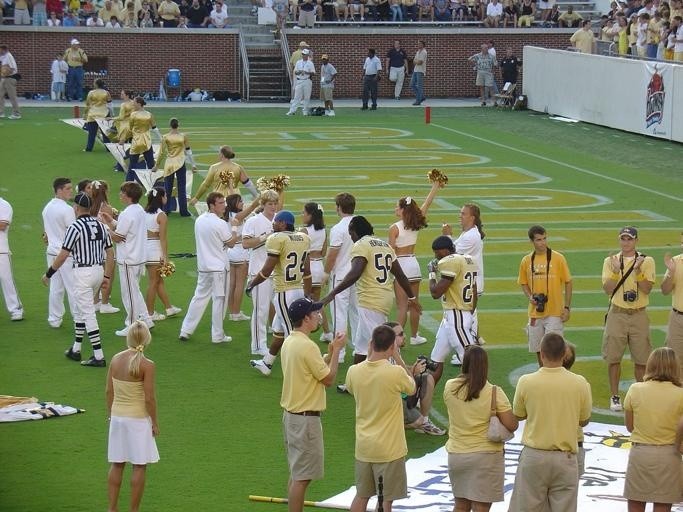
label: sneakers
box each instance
[609,394,623,411]
[320,331,485,365]
[80,356,106,367]
[64,347,81,361]
[249,358,272,376]
[414,418,447,437]
[361,96,426,110]
[94,291,270,355]
[286,108,337,117]
[337,384,347,392]
[56,96,83,103]
[8,112,22,119]
[10,308,23,321]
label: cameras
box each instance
[624,289,636,301]
[533,293,547,312]
[417,355,439,372]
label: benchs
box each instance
[276,1,683,33]
[0,14,36,26]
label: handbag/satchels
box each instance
[488,385,515,442]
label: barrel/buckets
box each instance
[166,69,181,87]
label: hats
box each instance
[69,192,92,208]
[619,226,638,239]
[300,41,309,47]
[71,39,80,45]
[274,210,295,225]
[322,53,328,60]
[288,297,324,321]
[302,48,309,55]
[432,235,453,250]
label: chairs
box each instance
[164,68,182,100]
[491,83,519,111]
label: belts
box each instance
[613,307,645,314]
[294,410,321,416]
[672,308,683,315]
